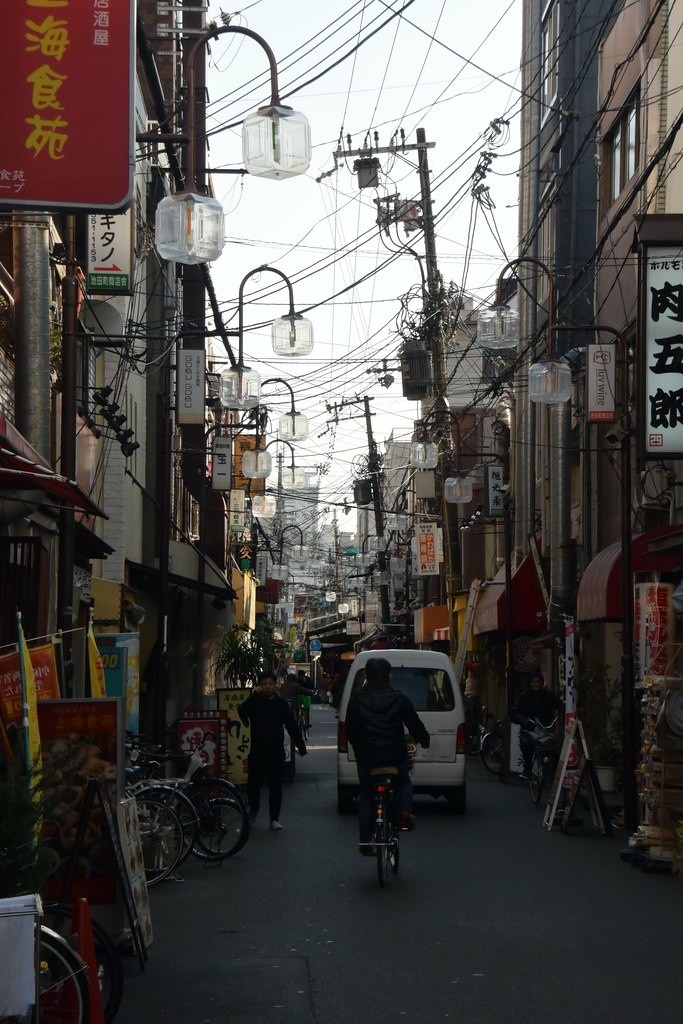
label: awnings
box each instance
[473,537,547,635]
[127,540,233,600]
[0,447,110,521]
[577,523,683,623]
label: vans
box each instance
[335,648,467,814]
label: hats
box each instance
[364,657,395,675]
[297,669,306,674]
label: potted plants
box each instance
[577,662,623,792]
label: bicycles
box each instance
[465,715,561,806]
[121,727,249,888]
[297,695,313,740]
[357,734,428,888]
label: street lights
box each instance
[474,255,639,836]
[411,408,519,730]
[151,22,312,571]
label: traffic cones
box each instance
[56,898,106,1024]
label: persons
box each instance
[279,673,314,708]
[344,657,430,855]
[237,671,307,831]
[298,670,316,728]
[510,673,564,781]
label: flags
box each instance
[87,624,107,698]
[21,632,43,845]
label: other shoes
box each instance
[269,819,284,831]
[523,768,530,777]
[398,813,416,831]
[247,813,259,826]
[359,842,374,856]
